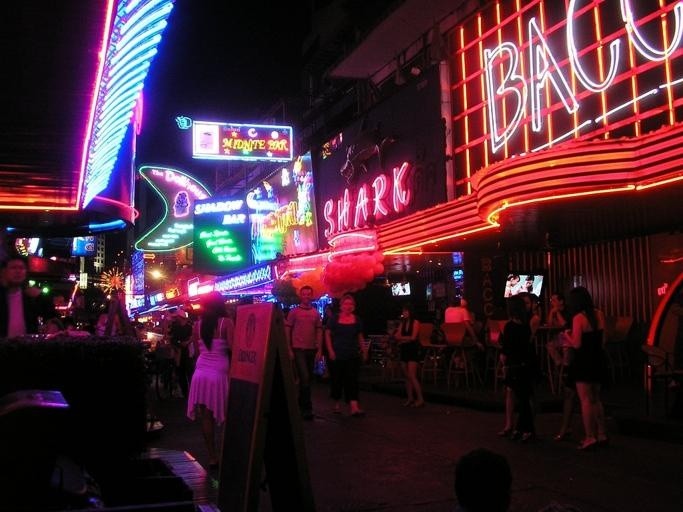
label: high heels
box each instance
[575,433,608,451]
[495,425,514,439]
[553,429,571,440]
[509,430,535,442]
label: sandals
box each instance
[206,399,424,469]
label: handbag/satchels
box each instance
[494,359,511,381]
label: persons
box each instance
[560,286,609,450]
[0,253,123,343]
[453,446,514,511]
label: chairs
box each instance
[358,311,635,396]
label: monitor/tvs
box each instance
[391,282,412,296]
[502,272,544,300]
